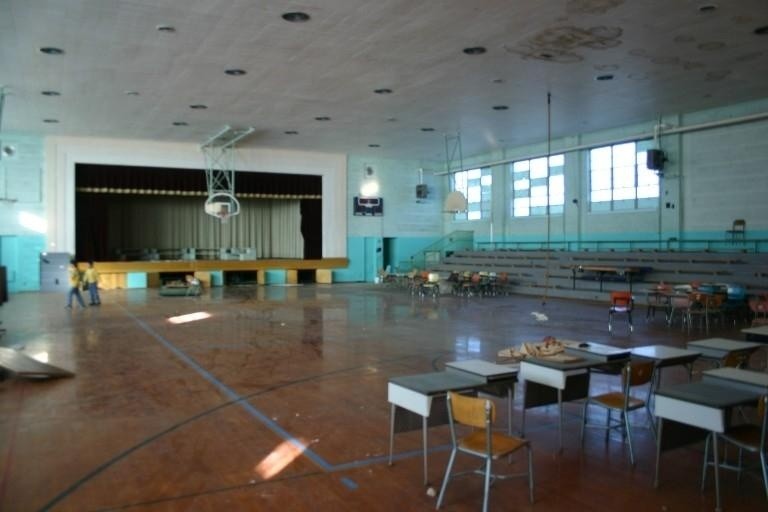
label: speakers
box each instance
[646,149,666,171]
[416,184,428,199]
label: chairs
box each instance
[605,278,748,334]
[607,292,636,334]
[384,269,511,302]
[436,391,534,512]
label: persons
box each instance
[64,259,87,310]
[83,260,101,306]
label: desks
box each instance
[579,360,658,465]
[686,336,763,366]
[630,344,699,386]
[385,372,484,480]
